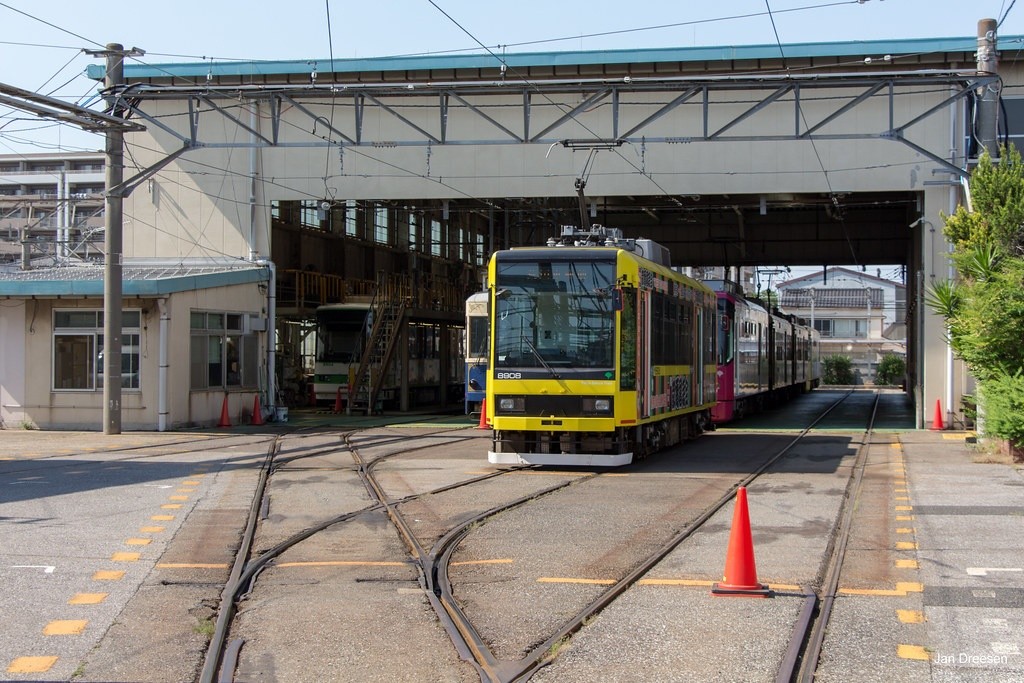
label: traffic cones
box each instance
[333,389,343,412]
[473,398,492,431]
[709,487,772,598]
[308,384,318,408]
[216,397,232,429]
[249,395,266,425]
[929,399,946,431]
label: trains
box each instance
[311,305,468,410]
[484,225,820,470]
[464,290,487,423]
